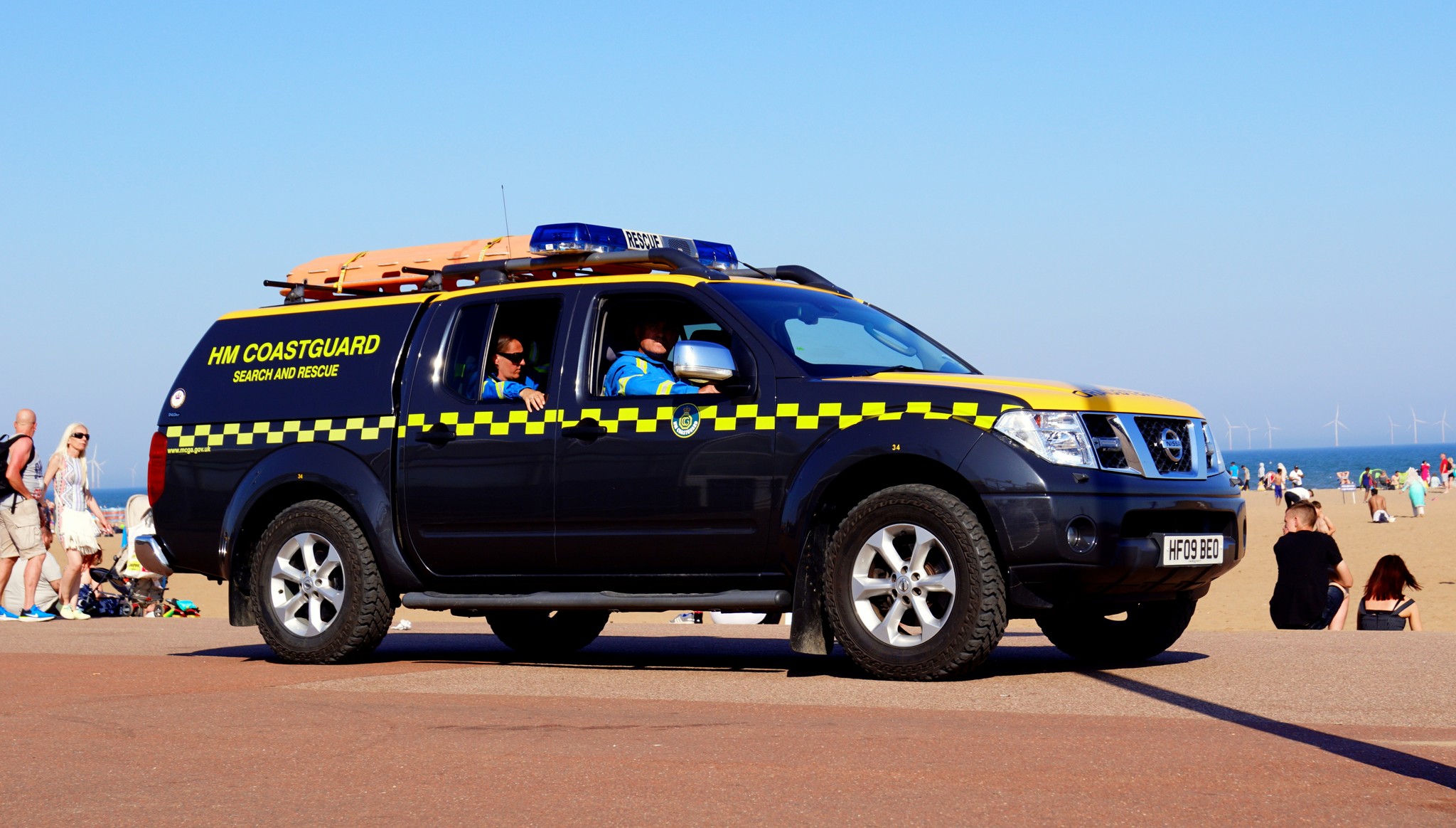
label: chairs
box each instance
[450,333,482,396]
[596,330,639,398]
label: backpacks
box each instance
[0,434,35,513]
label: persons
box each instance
[1225,461,1250,491]
[1269,500,1353,630]
[0,409,56,623]
[1257,462,1287,490]
[1379,453,1456,494]
[1399,467,1427,517]
[1368,489,1388,522]
[1312,501,1336,537]
[1357,555,1423,632]
[1284,487,1314,507]
[462,334,545,413]
[1266,468,1286,506]
[1360,467,1374,503]
[0,498,159,616]
[1336,471,1351,484]
[44,423,113,621]
[1289,465,1304,487]
[601,308,721,394]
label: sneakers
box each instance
[55,601,75,619]
[73,609,91,620]
[0,605,19,621]
[18,604,57,622]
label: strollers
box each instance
[76,494,171,618]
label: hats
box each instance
[1294,465,1298,469]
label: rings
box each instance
[530,397,535,400]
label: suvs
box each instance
[146,221,1251,680]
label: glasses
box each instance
[496,352,524,362]
[71,432,90,440]
